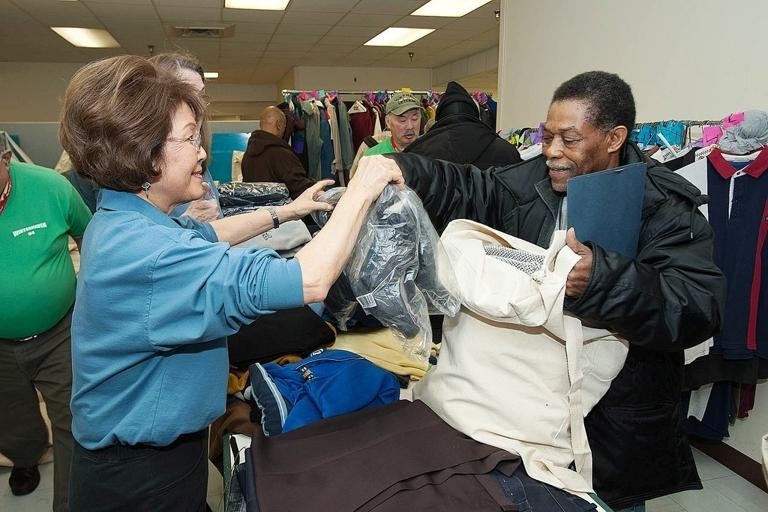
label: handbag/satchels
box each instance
[399,218,631,470]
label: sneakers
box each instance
[9,465,40,496]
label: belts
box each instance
[2,332,53,346]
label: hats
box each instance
[436,81,480,118]
[0,130,12,164]
[385,92,421,116]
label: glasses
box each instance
[165,131,203,152]
